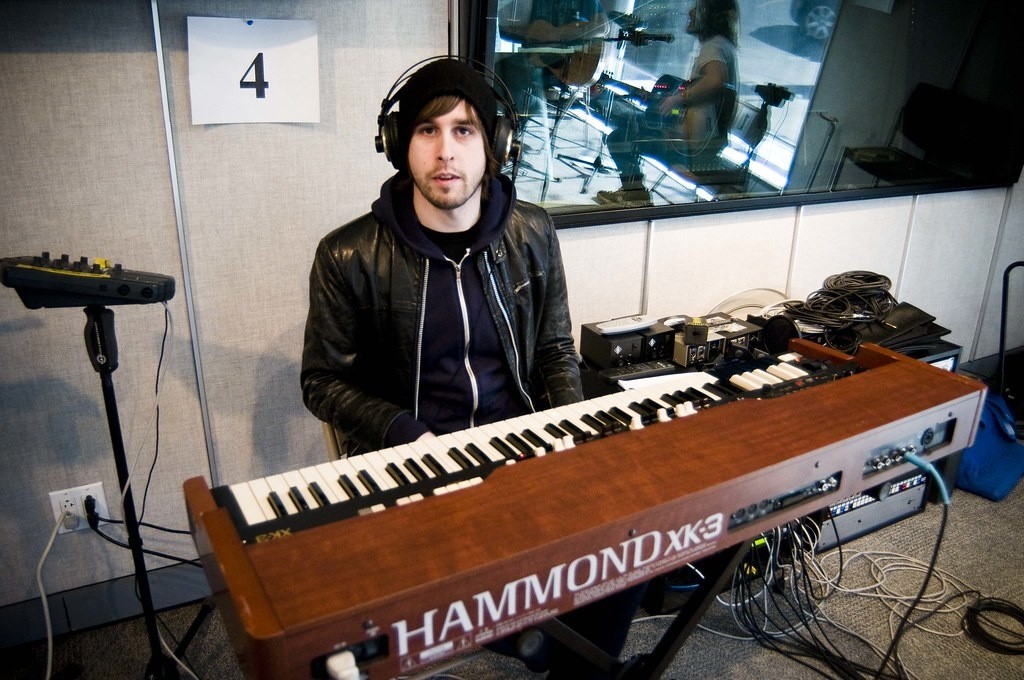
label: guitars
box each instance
[496,18,611,90]
[599,69,740,165]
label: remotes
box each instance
[599,359,675,381]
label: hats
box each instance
[395,58,499,148]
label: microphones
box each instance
[644,32,676,44]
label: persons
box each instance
[297,56,637,680]
[496,0,611,96]
[597,1,742,207]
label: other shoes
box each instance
[597,173,651,205]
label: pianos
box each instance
[176,325,991,680]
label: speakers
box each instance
[579,313,676,370]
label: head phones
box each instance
[375,54,524,173]
